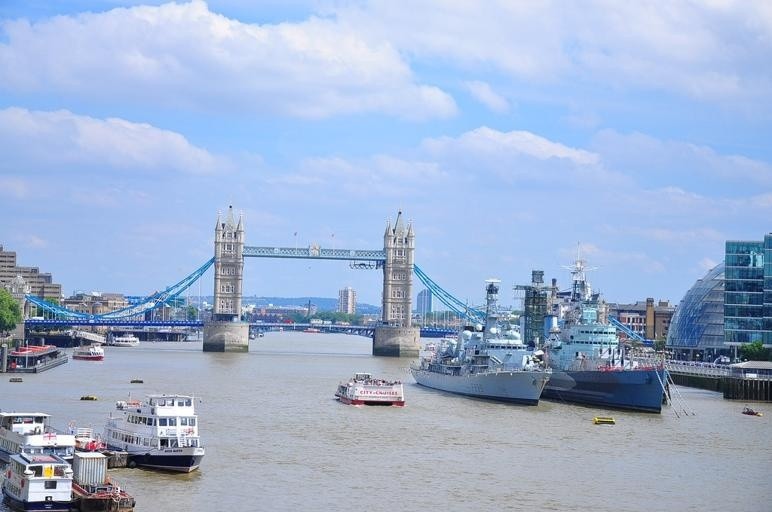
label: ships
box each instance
[410,278,553,405]
[542,239,670,414]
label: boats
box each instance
[335,373,405,406]
[743,409,762,417]
[73,342,104,360]
[104,392,204,473]
[0,411,135,512]
[114,333,139,346]
